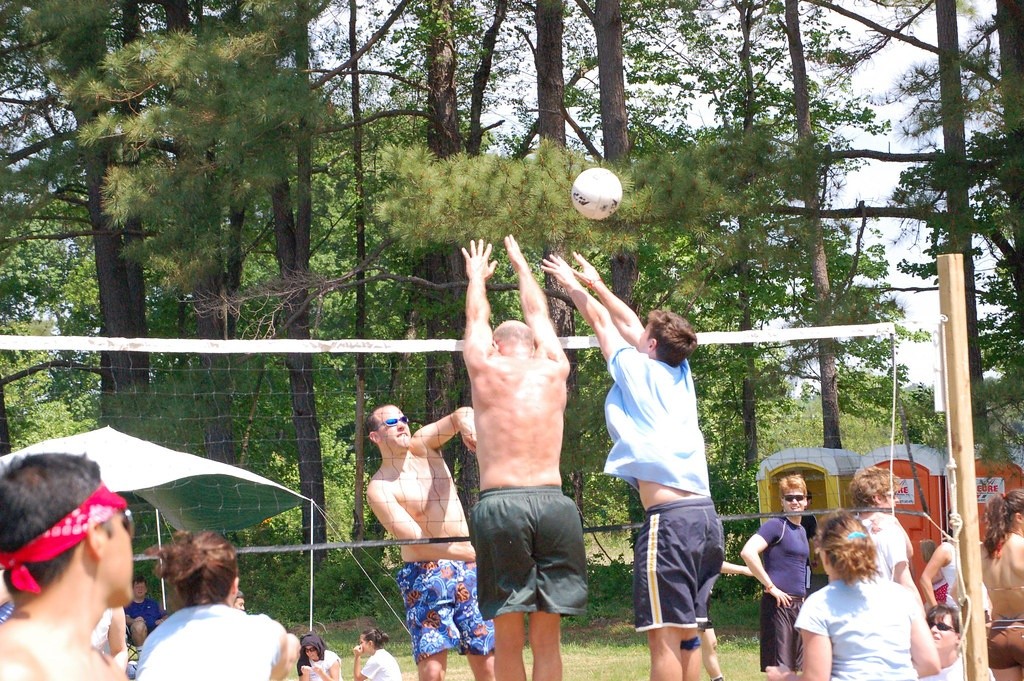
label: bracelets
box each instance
[766,584,774,592]
[588,277,605,285]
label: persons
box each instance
[848,466,927,619]
[461,232,587,681]
[740,474,810,680]
[353,628,402,681]
[126,575,169,661]
[135,531,302,681]
[980,489,1024,681]
[366,403,494,681]
[0,454,134,680]
[919,507,990,625]
[780,489,819,599]
[233,590,246,612]
[763,508,940,680]
[540,248,725,681]
[297,631,343,681]
[701,559,756,681]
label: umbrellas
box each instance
[0,426,311,535]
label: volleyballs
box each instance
[571,168,622,221]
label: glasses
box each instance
[303,647,316,653]
[372,416,408,433]
[926,621,953,630]
[102,508,134,535]
[783,494,804,501]
